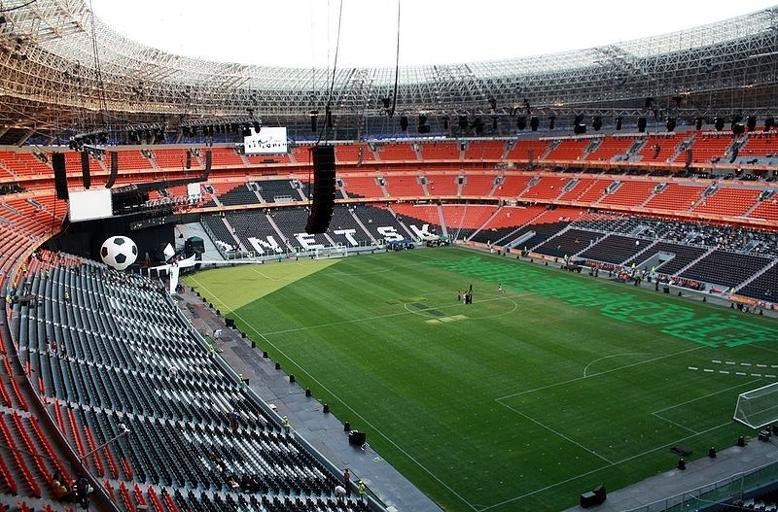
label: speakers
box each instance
[305,146,336,234]
[81,152,91,189]
[105,152,118,188]
[186,151,191,169]
[202,151,211,179]
[52,153,69,200]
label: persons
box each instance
[485,209,777,316]
[455,281,475,306]
[1,247,366,511]
